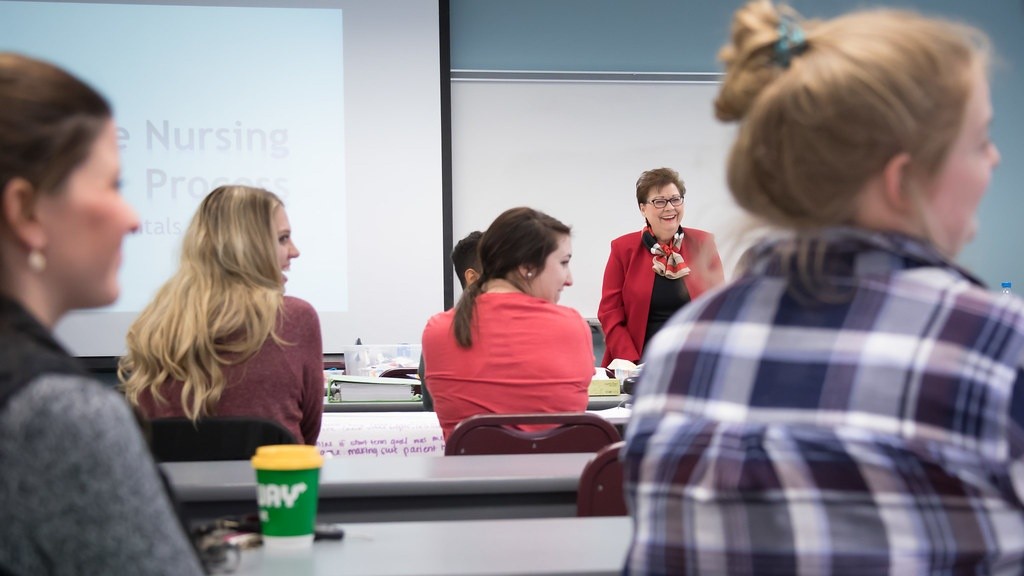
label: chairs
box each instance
[444,413,621,457]
[573,439,632,518]
[143,416,299,463]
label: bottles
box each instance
[1001,282,1013,295]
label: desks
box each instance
[212,515,635,576]
[315,409,621,521]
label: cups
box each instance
[250,444,323,549]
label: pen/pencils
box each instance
[218,519,345,541]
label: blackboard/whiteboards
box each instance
[448,69,796,337]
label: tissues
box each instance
[587,367,620,396]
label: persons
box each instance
[622,0,1024,576]
[0,53,210,576]
[118,185,324,445]
[419,231,485,411]
[421,206,595,441]
[597,167,726,378]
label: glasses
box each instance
[642,196,684,209]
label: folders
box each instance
[327,375,424,403]
